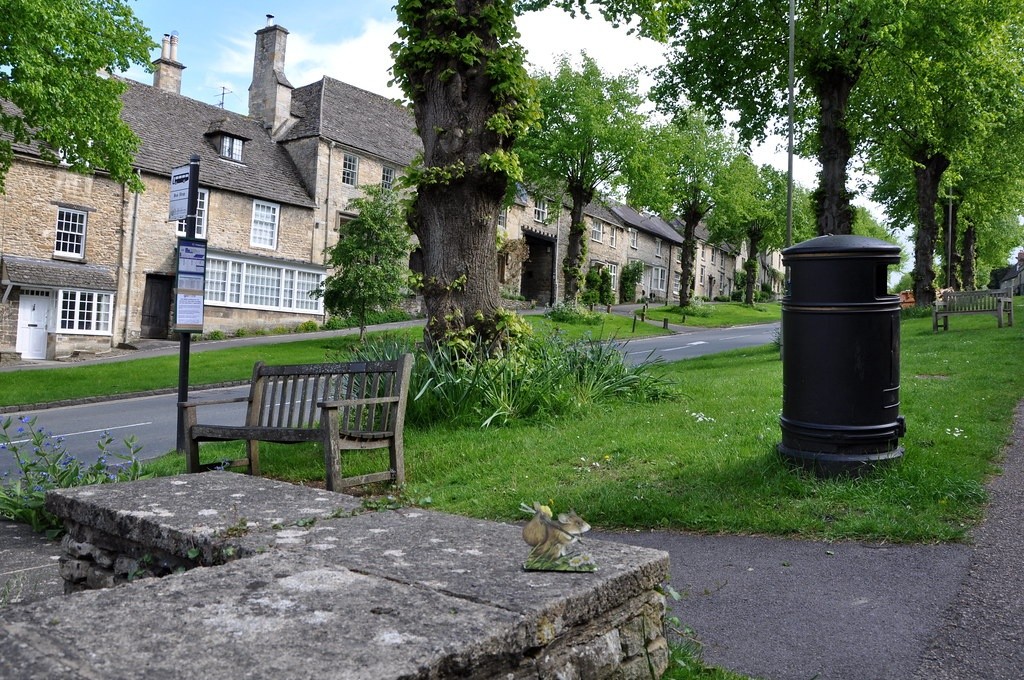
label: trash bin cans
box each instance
[780,234,908,467]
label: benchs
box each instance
[931,286,1015,332]
[177,352,417,494]
